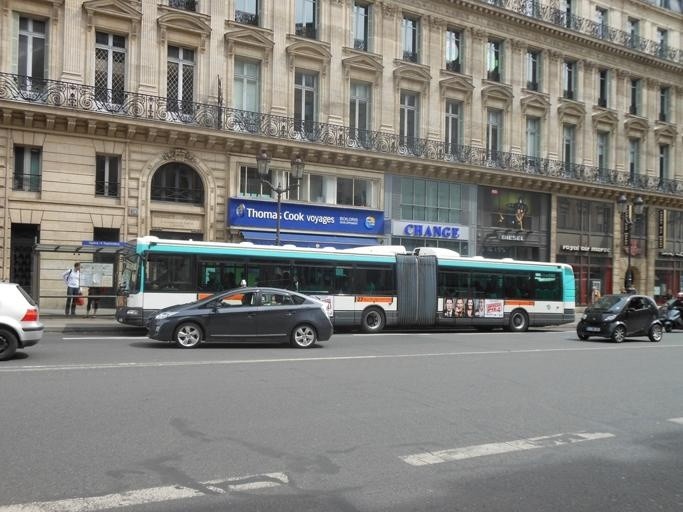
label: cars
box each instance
[146,286,334,349]
[576,293,683,343]
[0,281,44,361]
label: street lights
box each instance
[616,196,644,292]
[256,149,305,246]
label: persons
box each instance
[474,298,485,318]
[221,294,247,307]
[84,286,102,318]
[443,297,456,318]
[454,298,463,318]
[667,293,682,318]
[61,261,81,317]
[463,298,473,318]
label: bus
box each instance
[112,235,576,334]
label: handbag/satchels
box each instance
[76,292,85,306]
